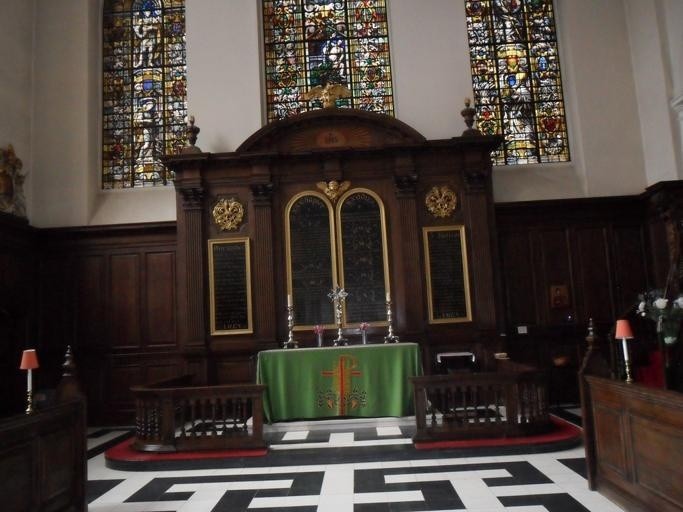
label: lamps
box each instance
[20,349,39,412]
[614,320,634,382]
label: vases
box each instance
[361,330,368,344]
[318,334,323,347]
[657,315,683,392]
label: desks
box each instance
[256,342,422,424]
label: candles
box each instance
[287,294,292,307]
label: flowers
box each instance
[635,289,683,322]
[359,322,371,330]
[313,325,326,335]
